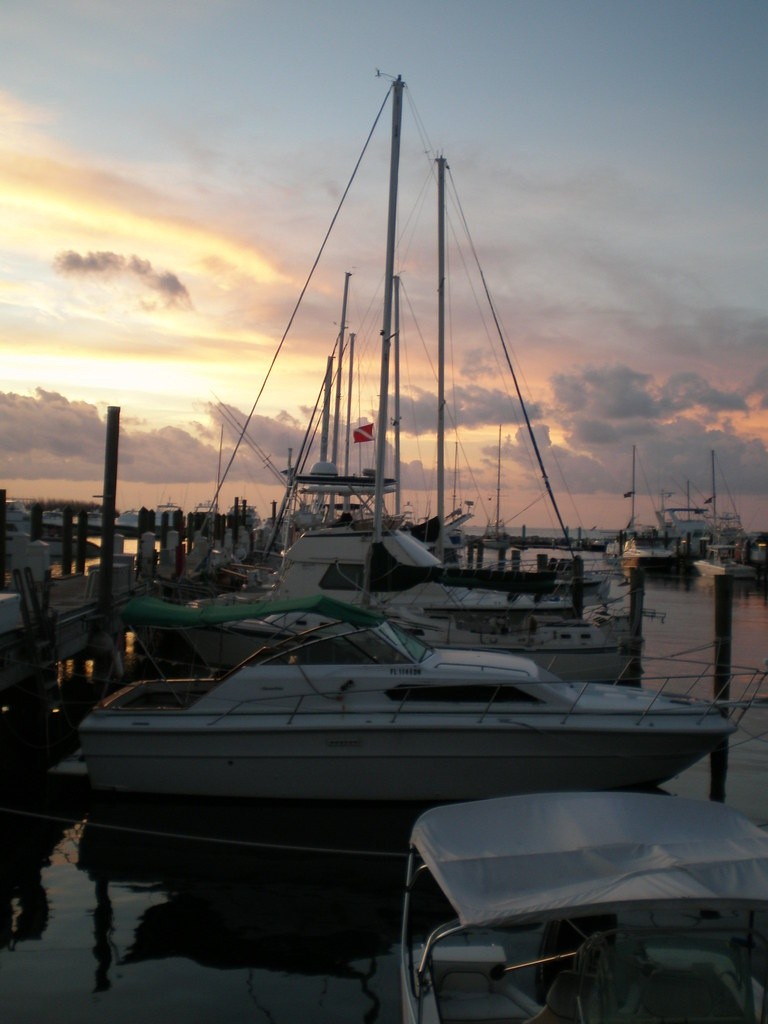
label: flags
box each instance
[354,423,375,443]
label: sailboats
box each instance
[1,73,768,1024]
